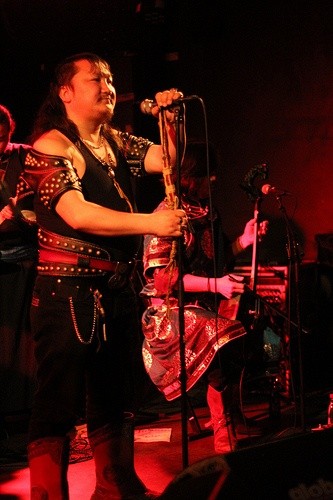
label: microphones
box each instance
[140,96,198,115]
[261,184,292,196]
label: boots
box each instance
[206,384,264,455]
[85,410,151,499]
[27,436,72,500]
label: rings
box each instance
[179,217,183,224]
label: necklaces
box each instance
[80,134,116,179]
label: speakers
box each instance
[158,428,333,500]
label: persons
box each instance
[24,54,186,499]
[0,107,34,422]
[142,142,269,453]
